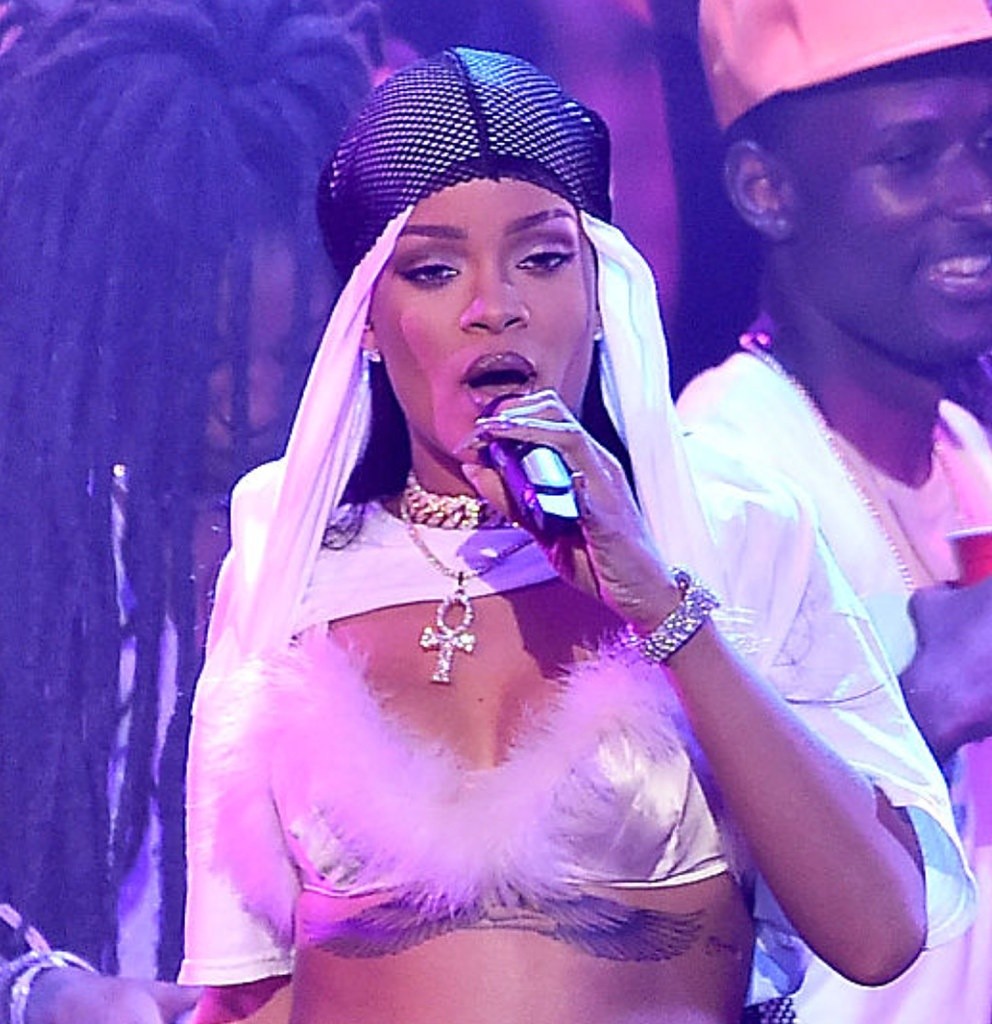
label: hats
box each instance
[696,0,992,141]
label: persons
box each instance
[0,0,377,1024]
[673,1,992,1024]
[181,48,983,1024]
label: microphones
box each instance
[477,393,580,535]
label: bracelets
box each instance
[0,946,100,1024]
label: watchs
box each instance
[627,564,721,665]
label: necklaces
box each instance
[398,468,540,686]
[738,329,975,591]
